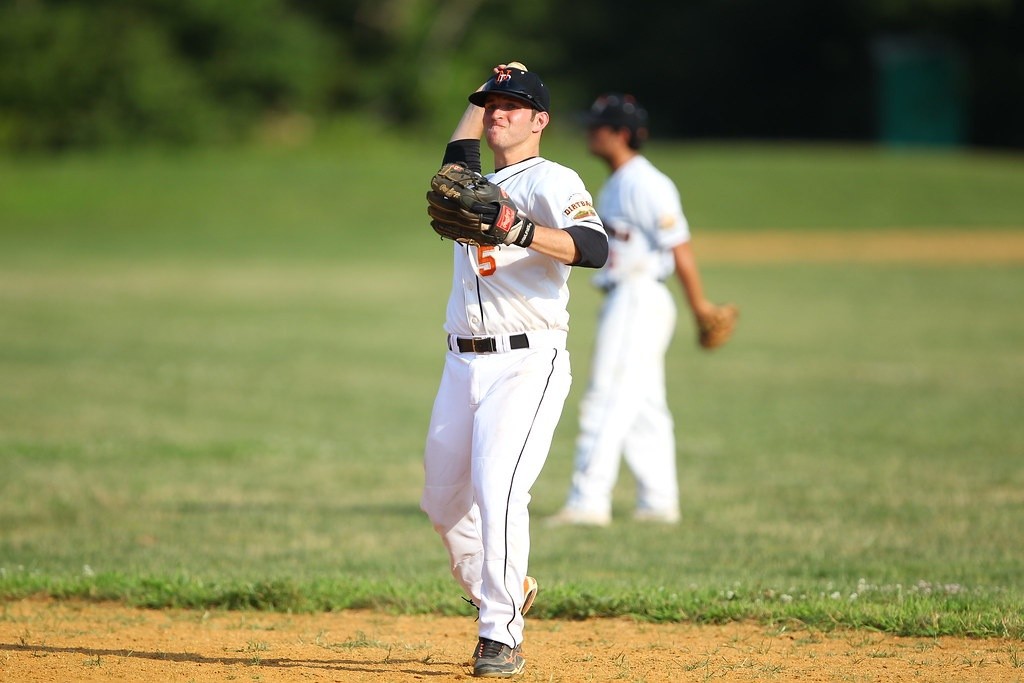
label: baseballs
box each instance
[505,61,528,72]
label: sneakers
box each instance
[519,575,538,615]
[474,637,525,677]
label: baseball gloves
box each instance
[701,305,738,347]
[426,162,518,246]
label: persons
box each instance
[419,61,608,677]
[546,89,733,527]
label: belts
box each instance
[445,331,532,354]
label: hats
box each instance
[574,89,654,132]
[468,67,551,112]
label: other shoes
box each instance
[548,506,688,528]
